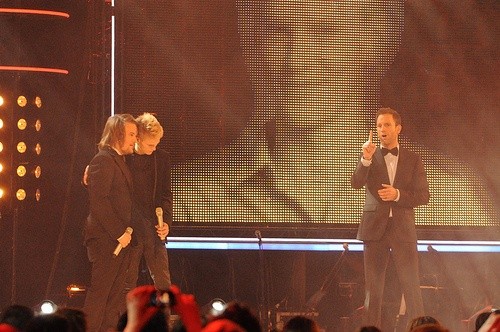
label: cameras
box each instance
[149,289,175,307]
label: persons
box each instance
[84,114,173,316]
[118,285,262,332]
[361,326,380,332]
[84,114,140,332]
[409,316,449,332]
[170,0,496,226]
[475,309,500,332]
[0,304,87,332]
[351,108,430,329]
[285,315,319,332]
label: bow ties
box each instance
[381,147,399,157]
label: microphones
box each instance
[155,207,165,241]
[112,227,133,256]
